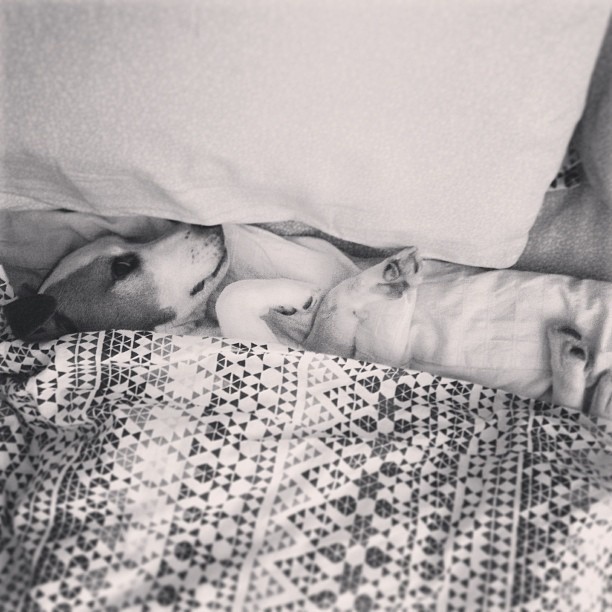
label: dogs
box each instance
[2,222,611,420]
